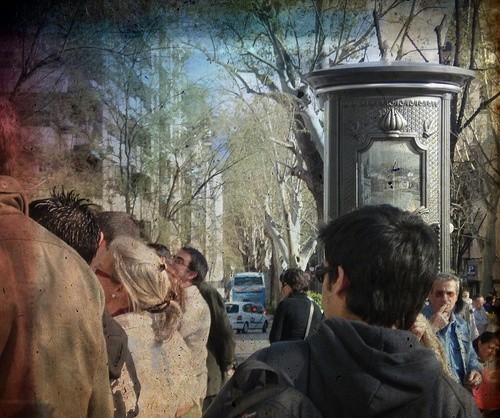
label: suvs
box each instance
[225,302,269,333]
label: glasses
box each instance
[282,281,288,288]
[170,256,191,272]
[314,264,339,283]
[93,266,112,279]
[432,291,457,298]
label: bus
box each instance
[230,272,265,307]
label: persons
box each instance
[89,211,141,270]
[144,243,171,265]
[167,248,210,418]
[28,186,142,418]
[95,236,201,417]
[422,271,483,394]
[269,267,324,344]
[201,203,483,418]
[0,126,116,418]
[453,287,500,418]
[194,281,235,418]
